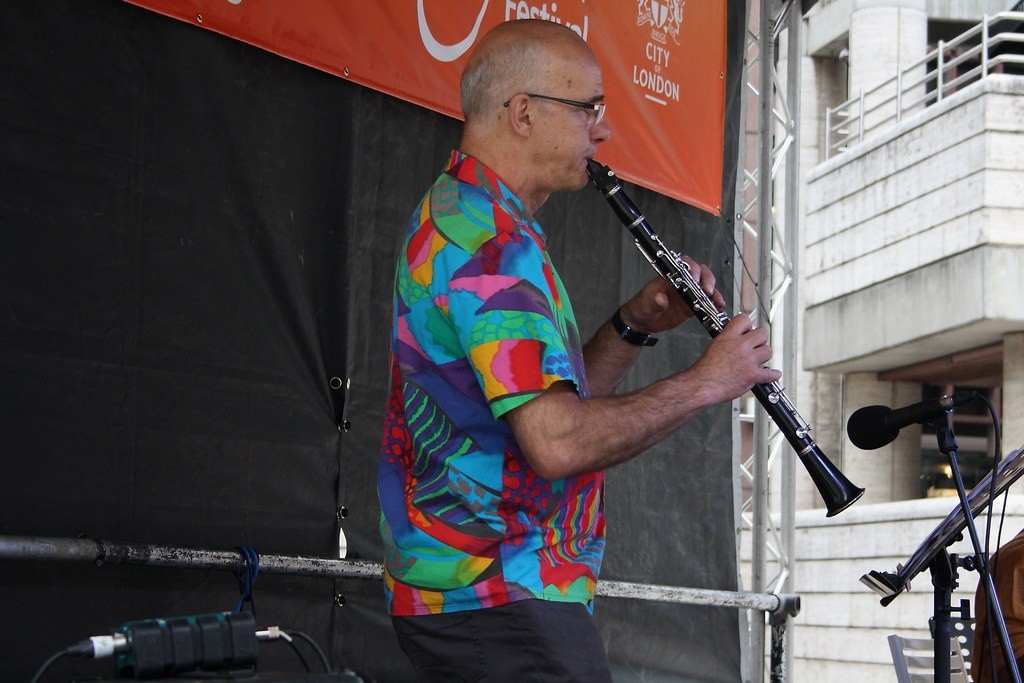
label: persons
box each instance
[969,528,1024,683]
[375,18,782,683]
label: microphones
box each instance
[847,390,979,450]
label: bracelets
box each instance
[610,306,659,347]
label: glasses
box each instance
[504,94,606,125]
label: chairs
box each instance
[887,598,975,683]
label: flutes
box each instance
[584,154,869,519]
